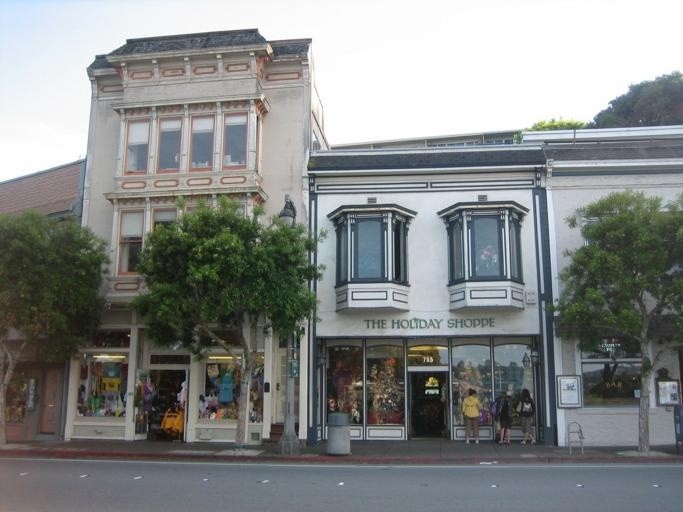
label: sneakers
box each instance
[466,439,470,444]
[521,440,527,445]
[531,439,537,445]
[475,439,479,444]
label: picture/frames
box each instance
[656,378,680,406]
[555,374,582,409]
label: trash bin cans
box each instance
[328,413,351,456]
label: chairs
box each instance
[567,422,584,456]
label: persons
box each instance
[461,387,482,444]
[497,390,515,444]
[515,389,536,445]
